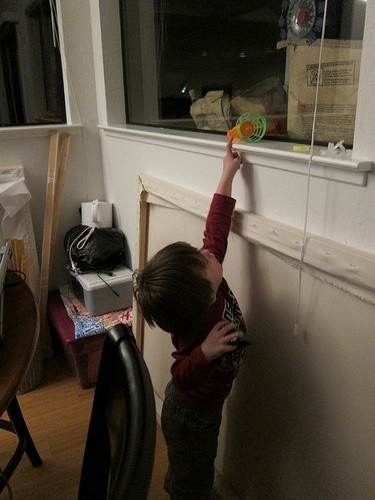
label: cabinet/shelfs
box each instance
[47,286,133,390]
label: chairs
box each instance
[77,319,157,500]
[0,267,42,500]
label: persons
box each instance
[131,134,248,500]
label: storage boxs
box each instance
[65,263,136,317]
[276,38,363,146]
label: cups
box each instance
[81,200,113,228]
